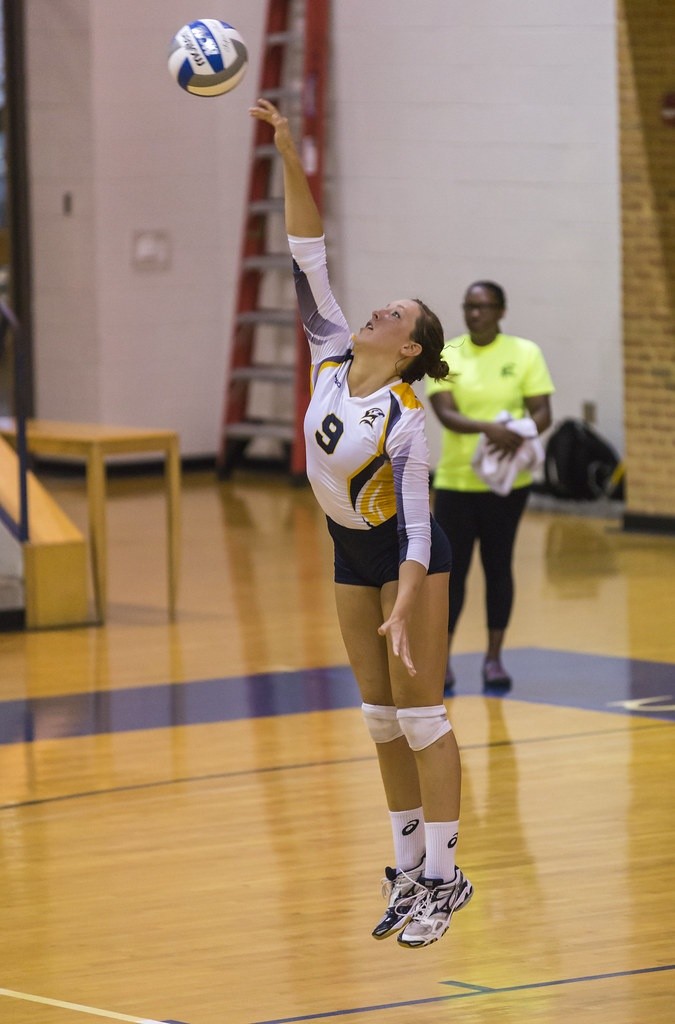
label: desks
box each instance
[0,418,179,624]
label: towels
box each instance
[470,411,546,496]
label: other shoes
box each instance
[482,667,512,693]
[444,677,455,691]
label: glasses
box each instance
[463,302,499,312]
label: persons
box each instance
[426,280,554,690]
[248,99,474,949]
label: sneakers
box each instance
[395,866,474,948]
[372,852,427,940]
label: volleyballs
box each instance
[168,18,250,98]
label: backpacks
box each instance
[545,417,620,499]
